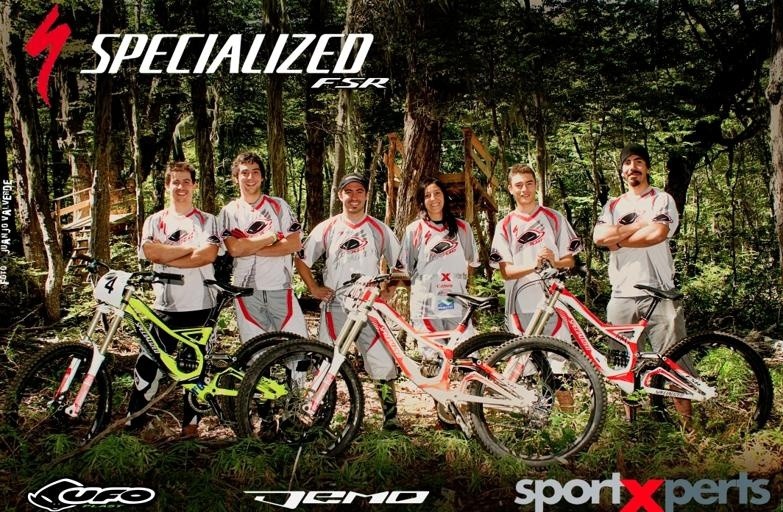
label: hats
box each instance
[619,143,650,167]
[339,174,367,193]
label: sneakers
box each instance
[182,424,198,439]
[382,420,409,442]
[255,420,278,441]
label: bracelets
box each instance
[617,243,621,248]
[269,233,278,244]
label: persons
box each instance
[293,173,403,431]
[489,163,582,414]
[397,176,481,429]
[217,152,310,443]
[592,143,696,434]
[123,161,228,438]
